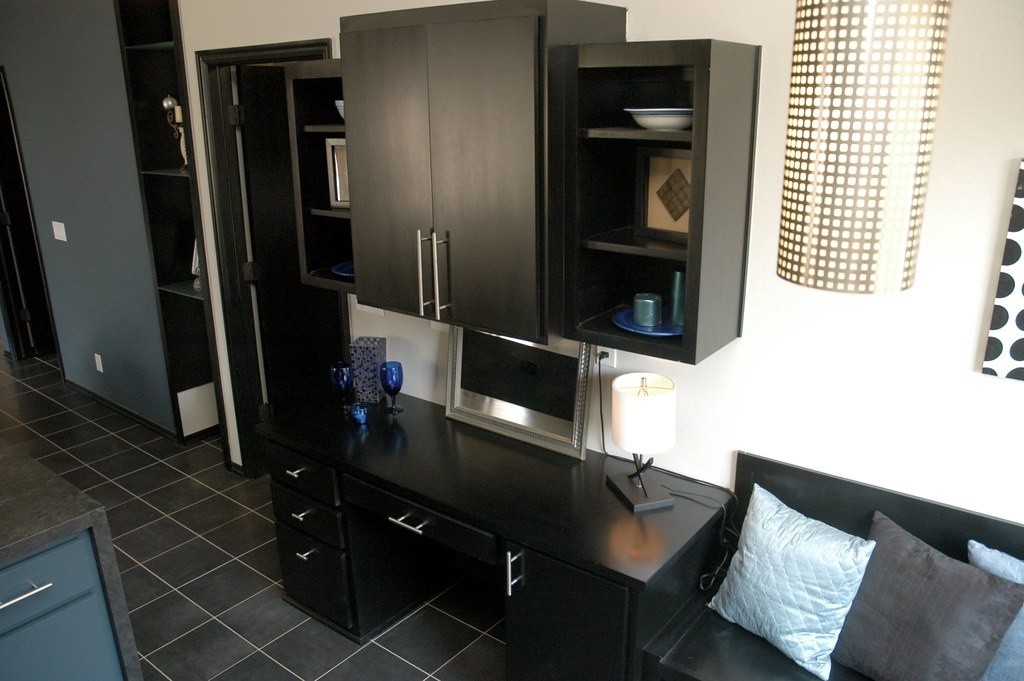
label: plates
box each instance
[612,306,686,336]
[332,263,354,276]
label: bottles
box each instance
[670,271,686,326]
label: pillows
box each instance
[967,539,1023,680]
[706,483,877,681]
[830,510,1023,680]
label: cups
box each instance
[351,402,370,424]
[634,294,661,327]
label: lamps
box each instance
[774,0,952,297]
[605,372,680,512]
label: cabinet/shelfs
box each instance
[281,57,357,298]
[563,37,763,365]
[339,0,627,349]
[111,0,233,472]
[0,436,144,681]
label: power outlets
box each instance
[93,352,104,373]
[596,345,618,369]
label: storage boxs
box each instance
[349,337,387,404]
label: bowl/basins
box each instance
[335,101,345,120]
[624,108,694,130]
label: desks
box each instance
[253,377,739,681]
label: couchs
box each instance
[644,448,1024,681]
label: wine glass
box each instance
[331,363,353,414]
[380,362,404,414]
